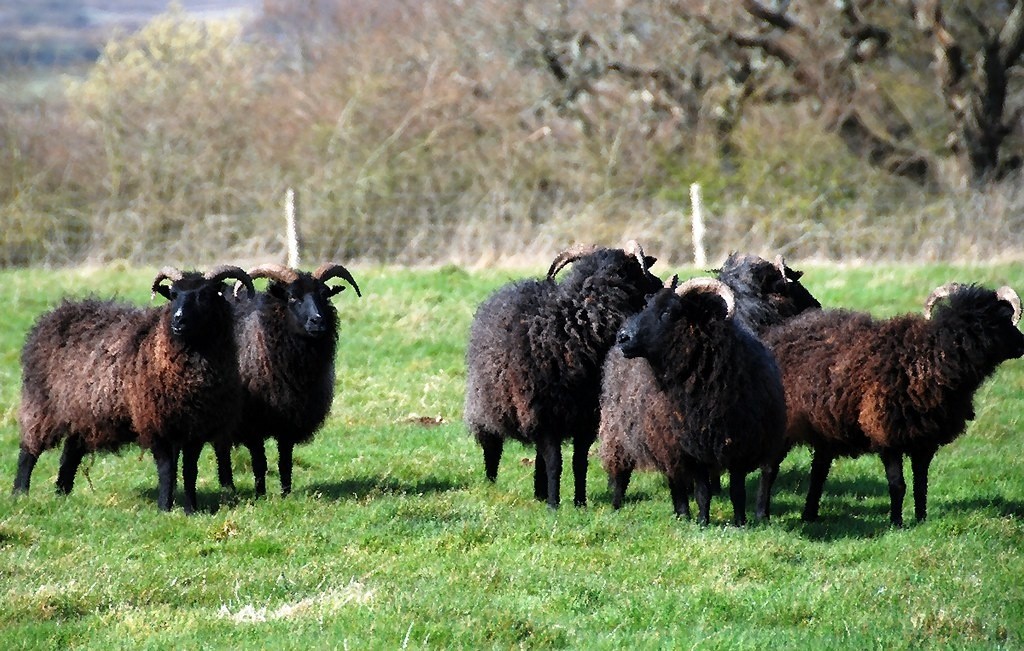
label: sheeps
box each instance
[466,243,1023,533]
[10,263,363,509]
